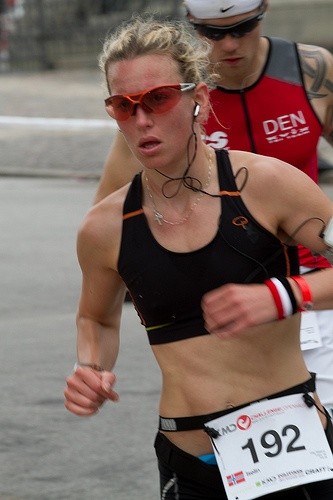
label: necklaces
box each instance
[145,141,211,225]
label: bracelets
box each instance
[262,276,315,322]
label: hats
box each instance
[186,0,262,19]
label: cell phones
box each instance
[322,219,333,248]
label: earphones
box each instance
[193,101,201,117]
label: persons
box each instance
[91,0,333,421]
[61,19,333,500]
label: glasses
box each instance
[104,83,196,121]
[189,12,263,41]
